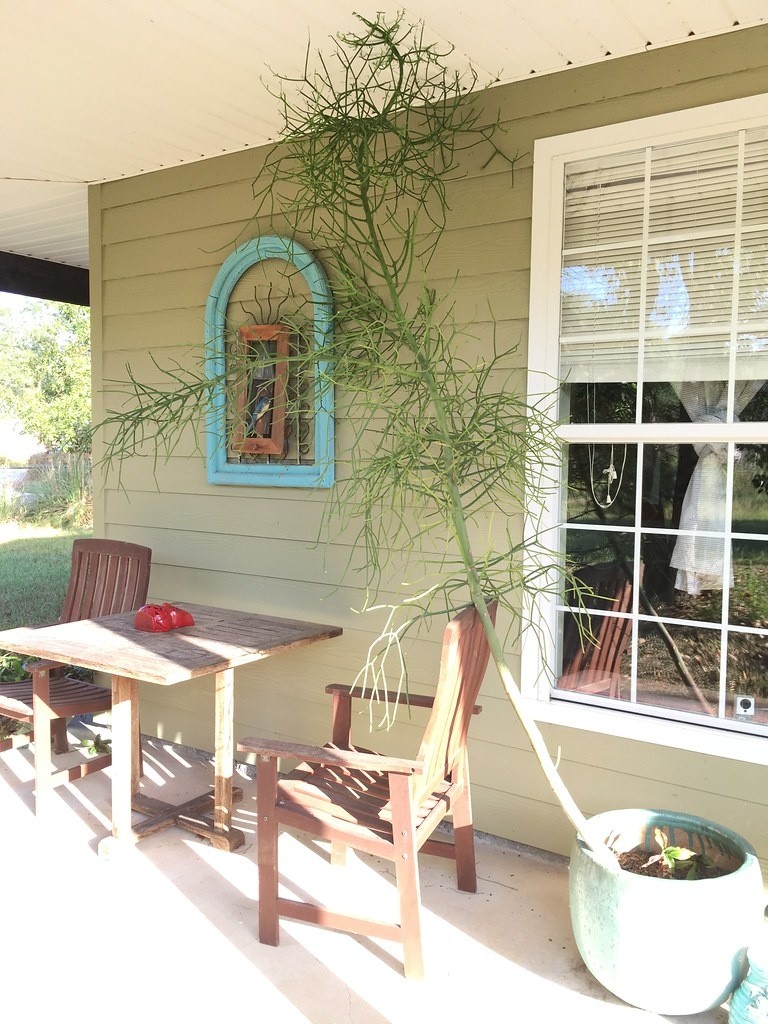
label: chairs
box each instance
[0,538,152,787]
[235,596,498,981]
[559,562,643,699]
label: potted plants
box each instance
[64,5,765,1013]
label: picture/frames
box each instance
[231,324,289,453]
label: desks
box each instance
[0,601,343,858]
